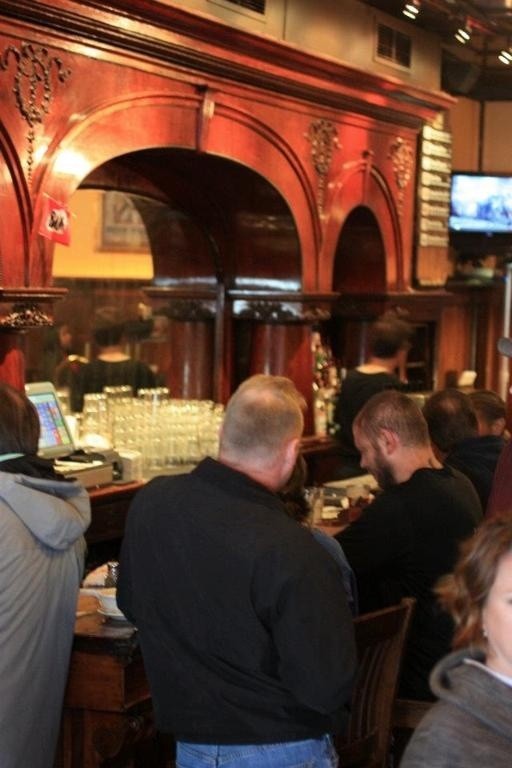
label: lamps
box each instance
[403,0,512,65]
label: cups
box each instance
[98,588,120,613]
[81,386,227,471]
[346,485,370,508]
[307,487,323,523]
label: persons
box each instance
[399,513,512,766]
[1,307,155,408]
[332,389,482,703]
[423,389,508,515]
[0,384,91,767]
[333,307,414,477]
[279,455,356,616]
[115,374,360,767]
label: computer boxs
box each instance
[53,458,112,489]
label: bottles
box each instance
[310,331,346,442]
[105,560,120,589]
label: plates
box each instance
[94,608,128,622]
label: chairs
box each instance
[353,598,418,768]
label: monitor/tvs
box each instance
[448,170,512,246]
[22,381,76,460]
